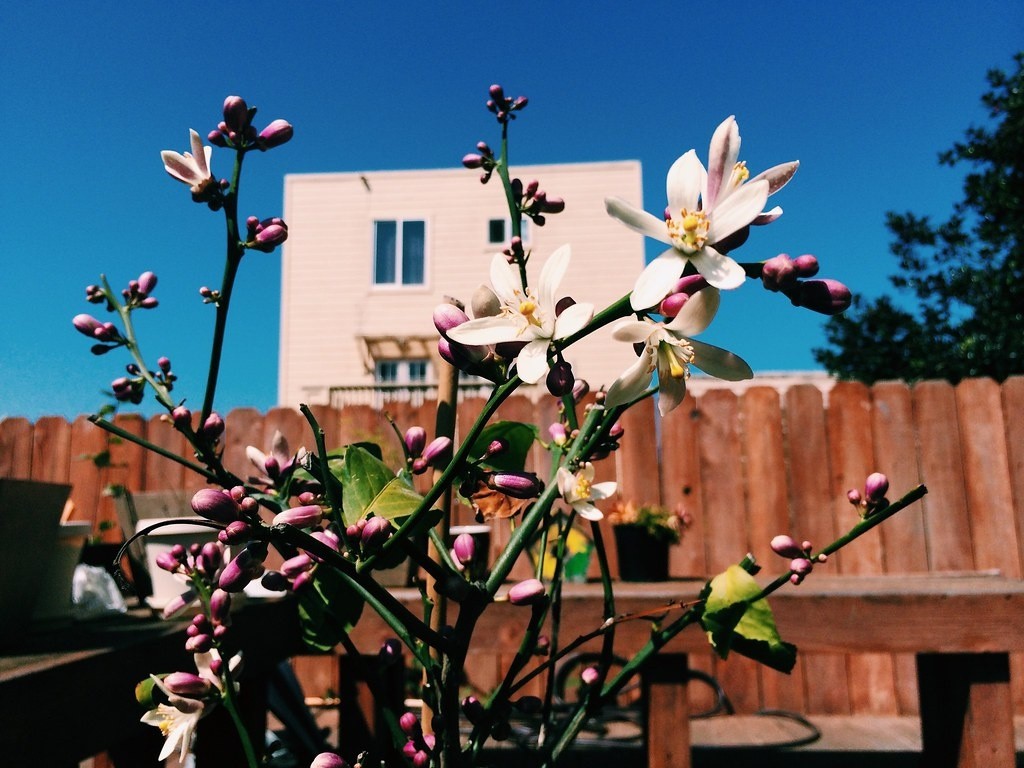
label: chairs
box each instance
[99,475,211,610]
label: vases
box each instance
[611,525,672,584]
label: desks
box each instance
[0,619,206,768]
[345,579,1023,768]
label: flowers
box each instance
[68,80,931,768]
[604,489,693,546]
